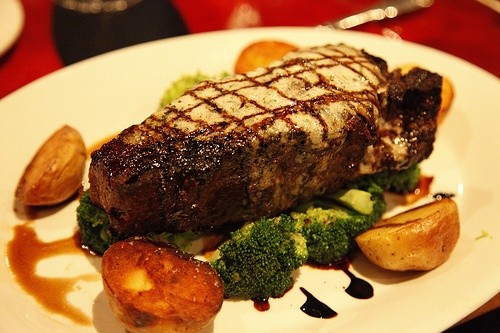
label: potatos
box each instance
[17,124,88,205]
[355,199,459,270]
[100,238,224,333]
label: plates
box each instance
[0,24,500,333]
[0,1,26,55]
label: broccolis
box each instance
[76,189,201,255]
[210,165,419,301]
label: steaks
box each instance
[88,42,443,237]
